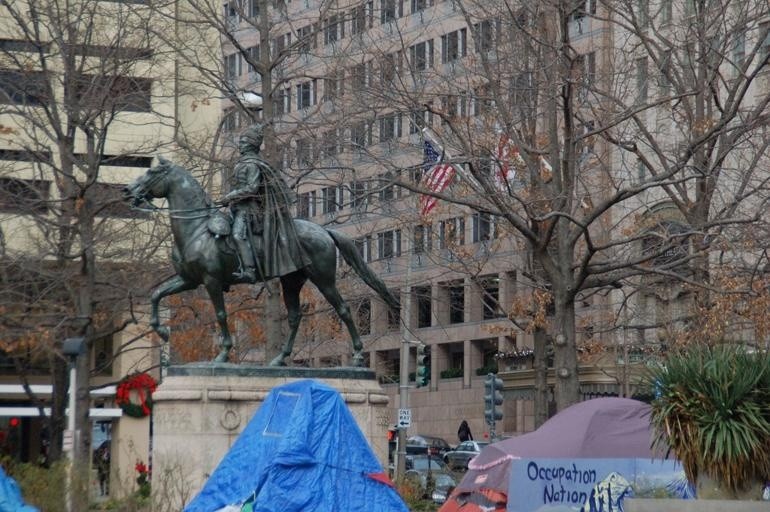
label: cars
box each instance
[389,434,491,504]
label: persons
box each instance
[92,444,110,496]
[220,125,313,285]
[458,420,474,442]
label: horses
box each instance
[119,151,405,367]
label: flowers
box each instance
[113,369,159,421]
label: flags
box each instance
[417,134,455,218]
[488,116,522,197]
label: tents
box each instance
[436,396,696,512]
[179,378,411,512]
[0,465,41,511]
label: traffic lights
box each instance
[416,343,431,388]
[484,372,503,420]
